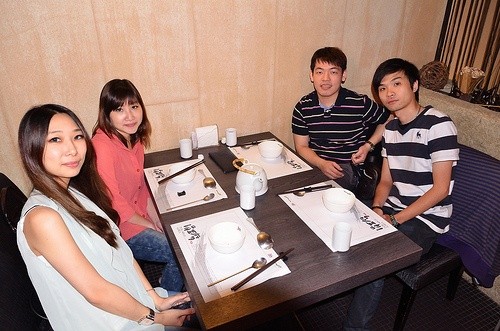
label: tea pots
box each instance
[232,157,268,195]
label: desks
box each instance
[144,131,423,331]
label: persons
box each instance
[344,58,459,331]
[90,78,185,299]
[292,46,395,194]
[17,104,195,331]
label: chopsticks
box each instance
[158,159,206,184]
[231,248,295,291]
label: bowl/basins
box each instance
[169,163,196,183]
[259,141,284,160]
[323,188,356,213]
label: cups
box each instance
[226,128,236,146]
[333,222,351,252]
[240,189,255,209]
[208,221,246,254]
[180,138,192,158]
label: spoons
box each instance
[207,257,267,287]
[198,168,216,189]
[166,193,214,210]
[247,219,274,249]
[293,187,332,196]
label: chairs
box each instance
[0,173,166,331]
[394,143,500,331]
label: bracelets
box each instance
[390,214,399,227]
[146,289,156,292]
[371,206,384,212]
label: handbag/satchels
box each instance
[352,160,377,195]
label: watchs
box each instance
[366,140,375,152]
[138,309,155,326]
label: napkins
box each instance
[195,125,218,148]
